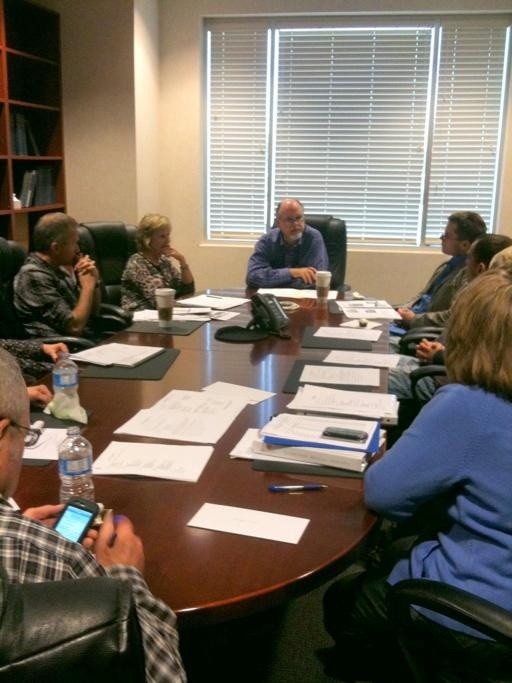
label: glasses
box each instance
[0,416,42,447]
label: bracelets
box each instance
[180,264,189,273]
[95,280,101,288]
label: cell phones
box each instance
[322,426,368,443]
[53,497,99,543]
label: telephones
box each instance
[246,294,293,340]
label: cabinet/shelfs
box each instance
[0,1,68,249]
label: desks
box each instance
[0,287,418,631]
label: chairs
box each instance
[75,222,139,320]
[272,214,348,290]
[0,238,126,351]
[371,578,511,681]
[1,579,143,683]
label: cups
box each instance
[315,271,332,303]
[154,288,176,328]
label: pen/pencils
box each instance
[268,484,328,493]
[207,296,222,299]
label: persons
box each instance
[245,196,330,290]
[12,211,102,378]
[0,344,189,682]
[323,270,511,682]
[120,210,196,312]
[388,207,512,400]
[0,338,70,409]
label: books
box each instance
[3,1,63,210]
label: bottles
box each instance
[58,427,94,506]
[52,352,80,420]
